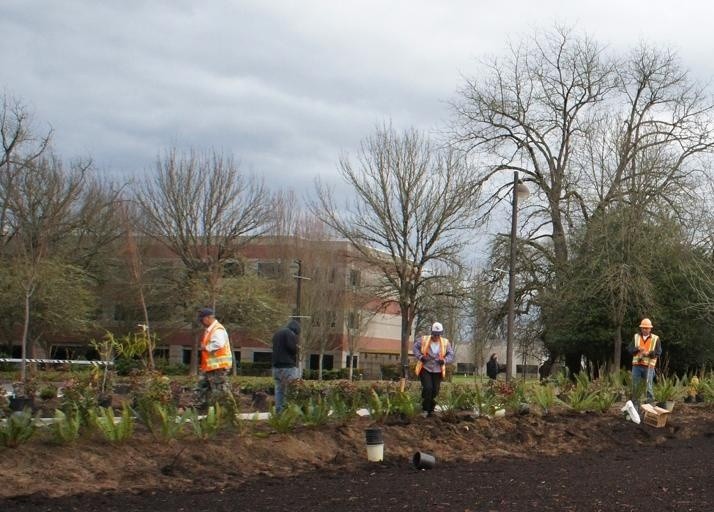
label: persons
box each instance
[626,318,662,404]
[413,322,454,418]
[271,320,301,415]
[192,308,239,418]
[486,353,500,380]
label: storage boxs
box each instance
[641,402,671,428]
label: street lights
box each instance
[506,172,530,380]
[289,261,302,367]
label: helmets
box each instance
[432,322,443,331]
[639,319,653,327]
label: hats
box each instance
[197,309,213,320]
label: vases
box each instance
[412,450,437,469]
[363,426,386,463]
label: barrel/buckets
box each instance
[412,451,436,468]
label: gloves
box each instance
[436,360,444,365]
[421,356,428,362]
